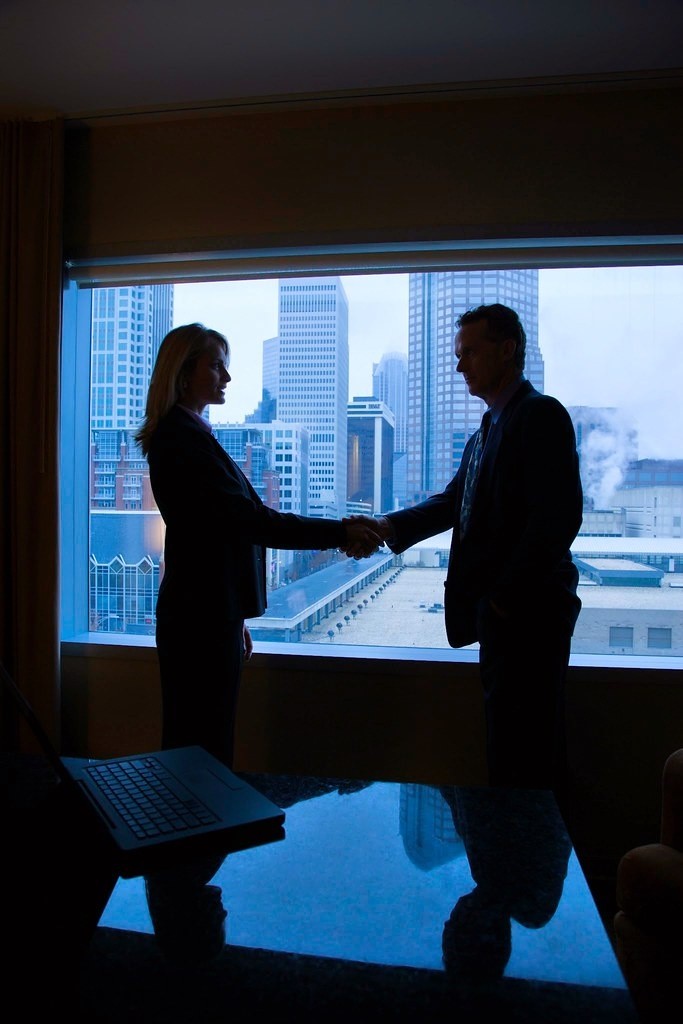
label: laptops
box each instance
[0,663,285,857]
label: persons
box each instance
[135,324,385,771]
[342,304,583,790]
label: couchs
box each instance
[613,745,683,1021]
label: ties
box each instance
[459,406,494,540]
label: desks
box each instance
[98,774,630,1006]
